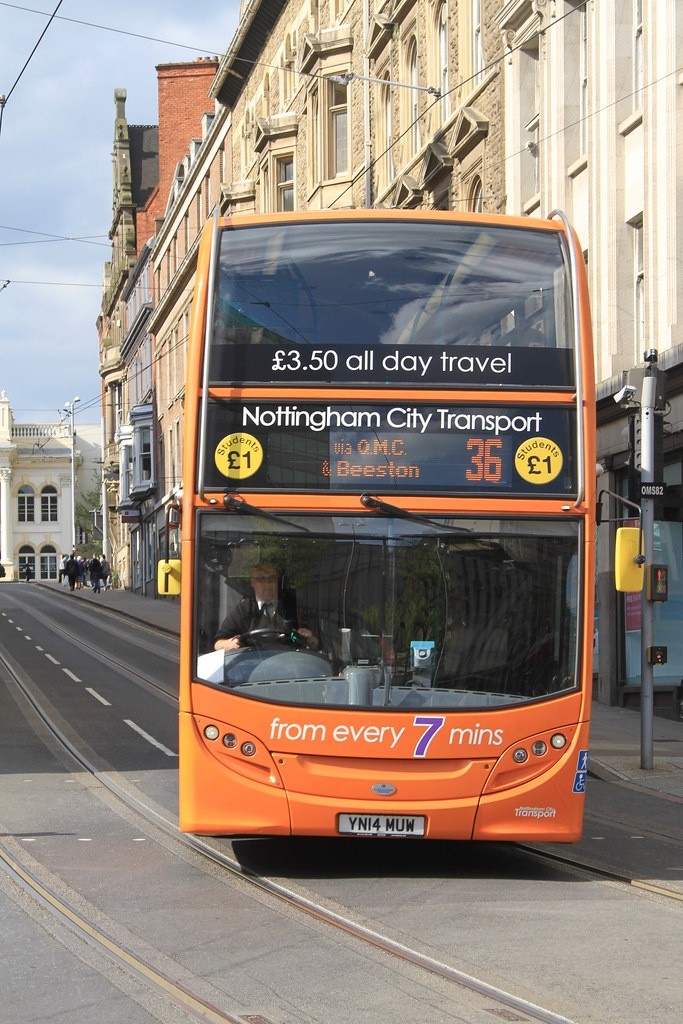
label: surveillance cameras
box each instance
[613,385,636,404]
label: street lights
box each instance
[63,395,81,557]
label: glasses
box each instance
[255,575,277,583]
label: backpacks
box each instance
[69,560,79,577]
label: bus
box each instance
[155,206,646,845]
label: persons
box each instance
[212,560,318,651]
[59,553,110,593]
[25,564,31,581]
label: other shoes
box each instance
[94,588,100,594]
[70,585,74,591]
